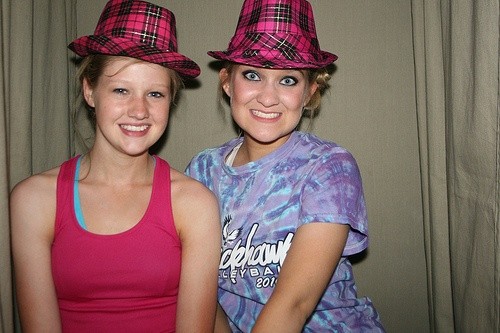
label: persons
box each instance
[183,0,387,333]
[10,0,222,333]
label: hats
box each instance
[207,0,338,69]
[68,0,201,82]
